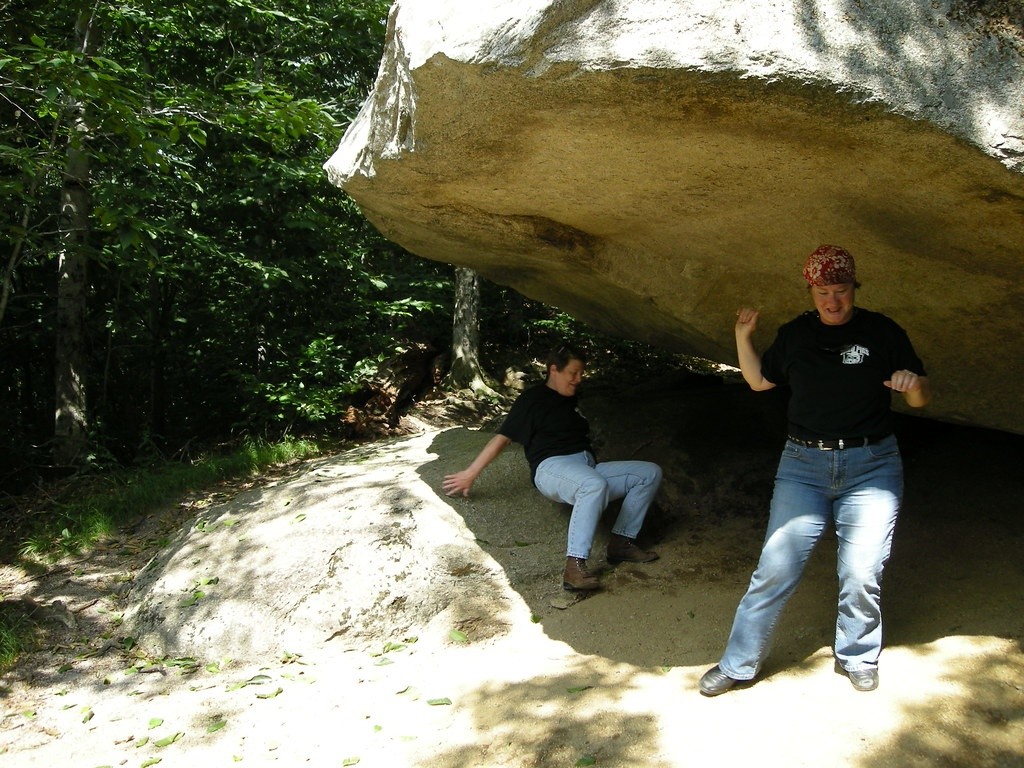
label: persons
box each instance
[442,343,663,590]
[699,243,933,697]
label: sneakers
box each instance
[564,557,601,590]
[605,536,659,563]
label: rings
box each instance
[907,373,912,376]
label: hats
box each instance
[802,245,856,286]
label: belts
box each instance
[788,426,896,450]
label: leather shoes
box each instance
[699,664,737,696]
[849,667,880,691]
